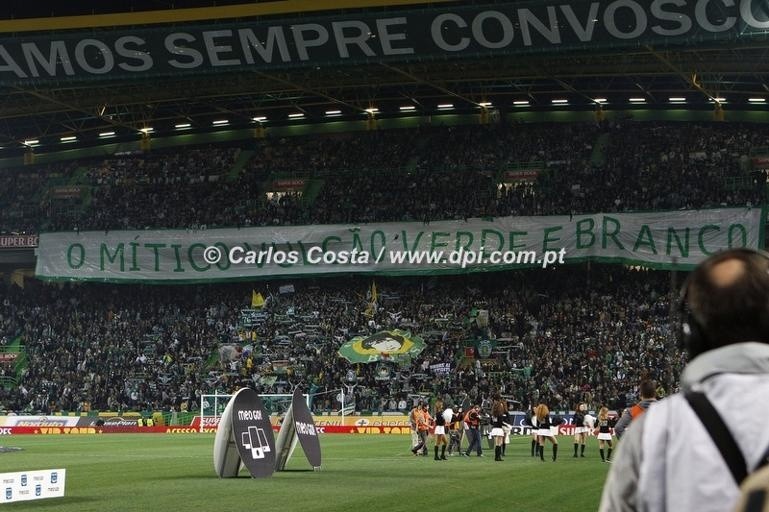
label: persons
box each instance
[598,248,769,512]
[411,379,658,462]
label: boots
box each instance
[600,448,612,464]
[412,440,559,462]
[572,443,585,457]
